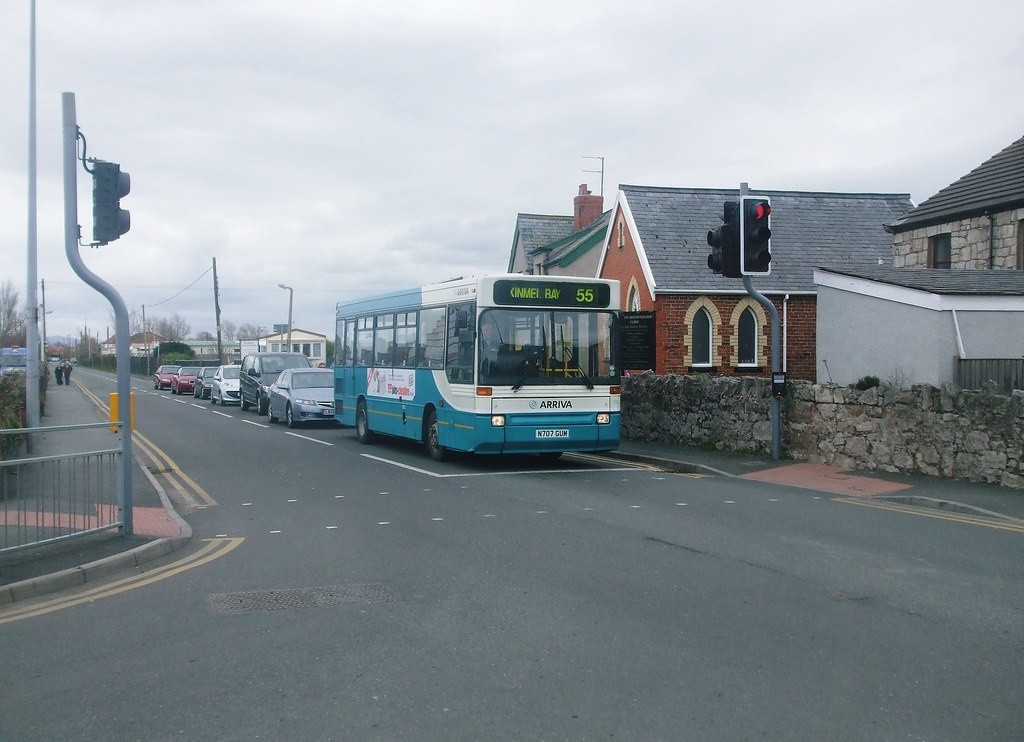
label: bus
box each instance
[334,273,621,462]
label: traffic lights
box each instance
[91,161,130,240]
[707,200,740,278]
[740,195,772,275]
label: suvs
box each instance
[240,351,311,416]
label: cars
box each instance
[267,368,334,428]
[153,365,182,390]
[0,345,26,378]
[211,364,241,406]
[48,356,60,362]
[59,357,78,370]
[170,367,202,395]
[192,367,219,400]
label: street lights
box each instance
[278,284,293,354]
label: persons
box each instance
[467,322,497,381]
[55,361,73,386]
